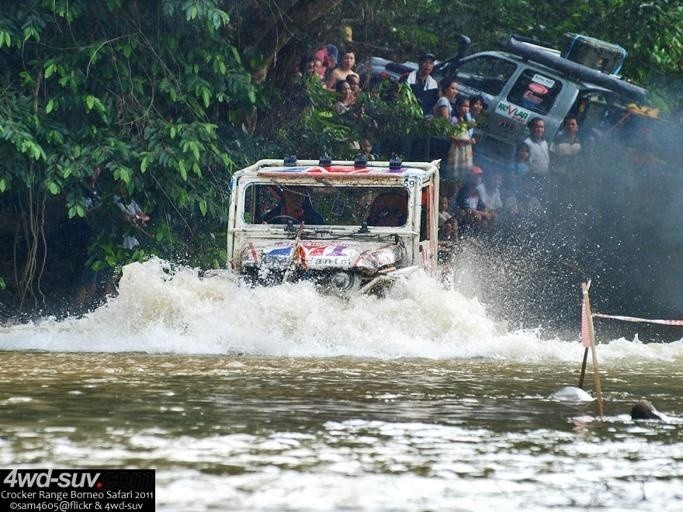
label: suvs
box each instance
[227,156,455,299]
[354,32,648,214]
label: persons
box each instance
[294,26,663,279]
[252,185,326,226]
[42,152,151,321]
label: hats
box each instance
[419,52,437,62]
[467,166,483,176]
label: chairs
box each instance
[365,194,406,226]
